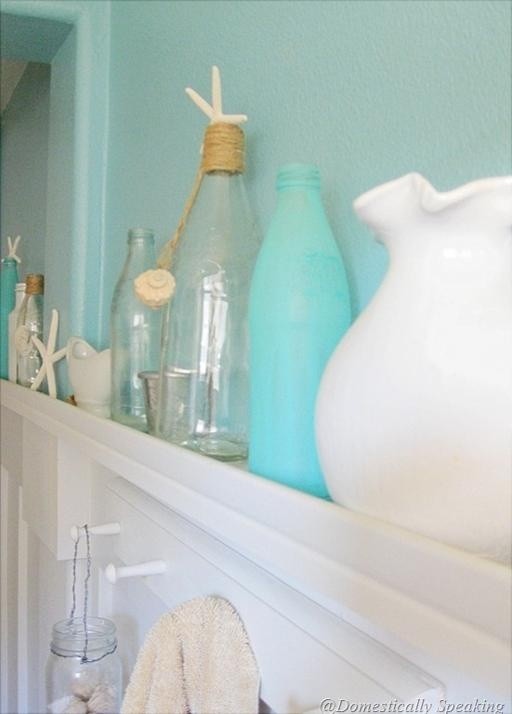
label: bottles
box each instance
[0,121,352,509]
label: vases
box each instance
[312,166,512,560]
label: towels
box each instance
[116,593,263,714]
